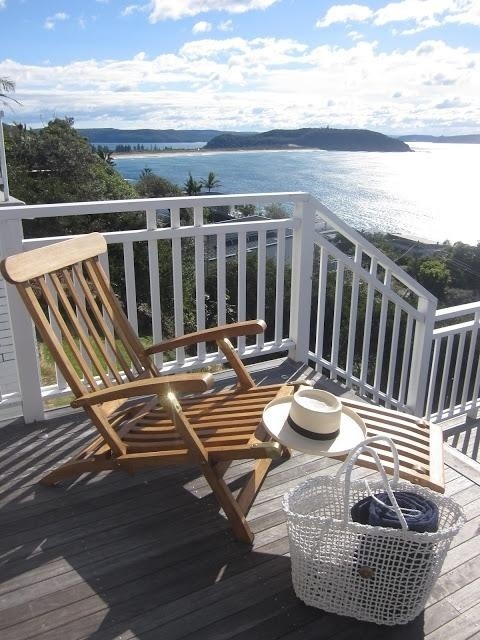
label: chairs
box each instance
[1,231,445,547]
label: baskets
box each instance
[282,434,468,626]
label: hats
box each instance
[262,388,366,457]
